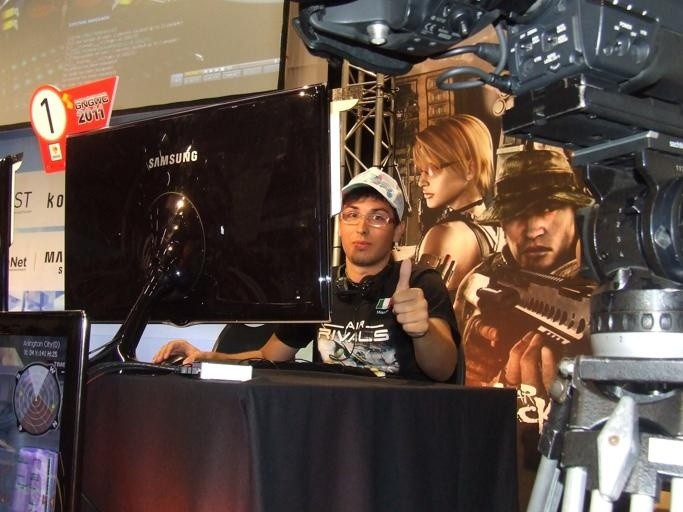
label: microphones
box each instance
[333,289,359,295]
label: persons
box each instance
[153,167,461,384]
[453,151,608,475]
[412,113,507,305]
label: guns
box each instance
[476,265,593,361]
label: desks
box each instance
[72,358,521,512]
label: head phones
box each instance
[335,254,394,302]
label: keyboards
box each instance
[240,358,382,381]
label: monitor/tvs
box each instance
[0,309,92,512]
[64,80,331,375]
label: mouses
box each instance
[160,354,188,365]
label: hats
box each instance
[475,149,593,227]
[340,167,407,225]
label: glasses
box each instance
[413,157,466,180]
[339,209,396,226]
